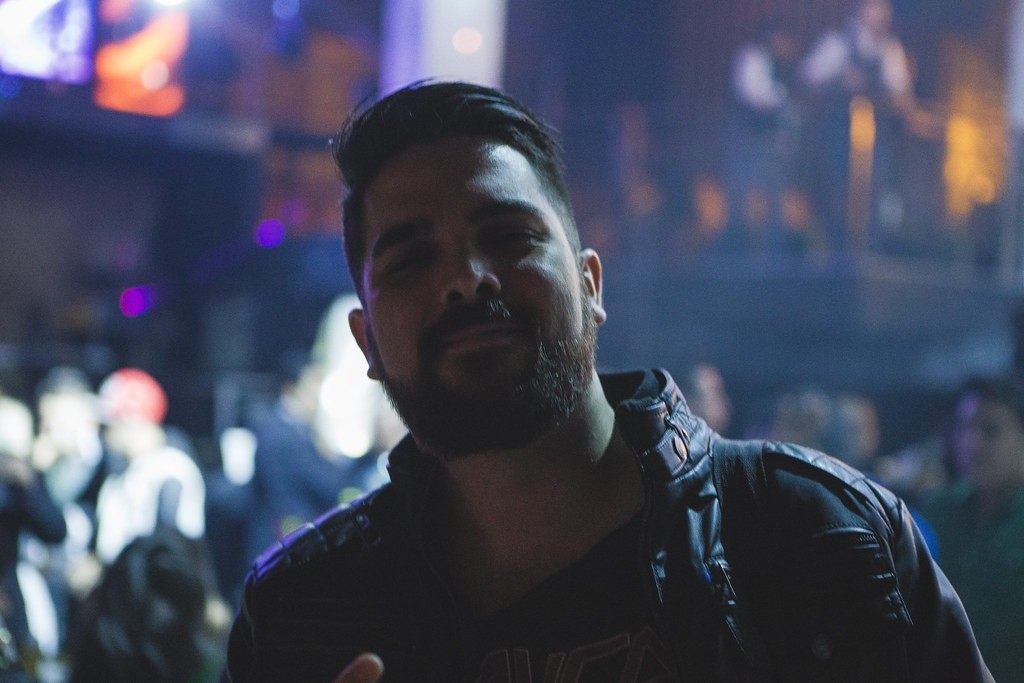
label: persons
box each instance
[0,287,411,682]
[675,358,1024,683]
[580,0,954,260]
[222,82,998,683]
